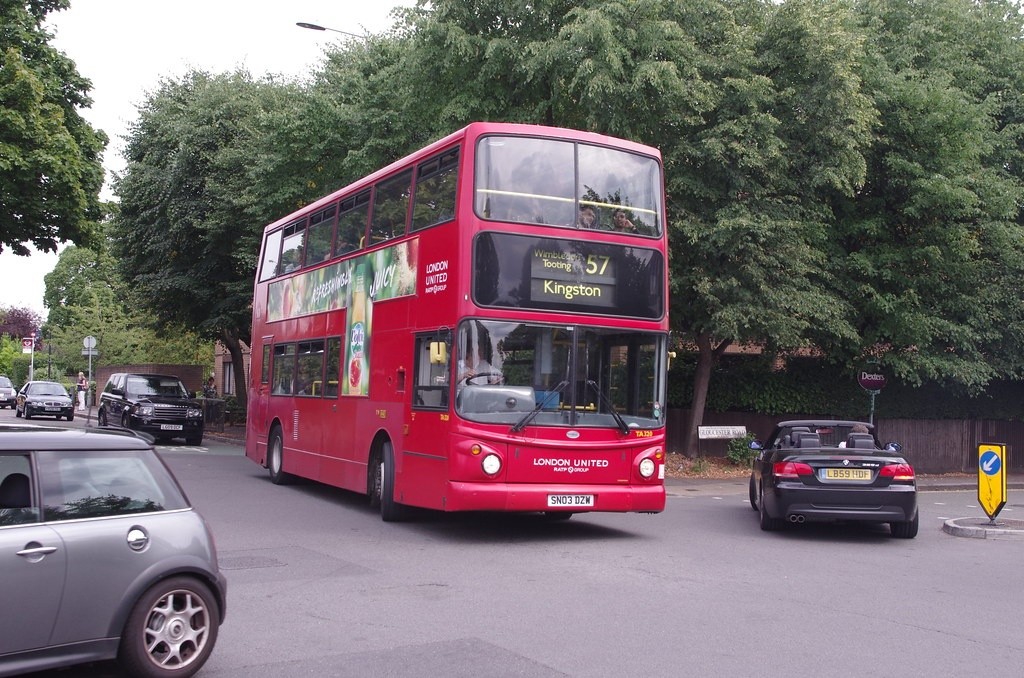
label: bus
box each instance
[245,122,677,523]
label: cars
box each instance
[748,419,918,538]
[1,423,227,678]
[0,375,17,410]
[15,380,75,421]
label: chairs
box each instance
[794,432,822,448]
[846,432,875,449]
[0,473,32,508]
[789,426,811,446]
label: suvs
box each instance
[98,372,204,445]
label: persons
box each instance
[285,235,354,273]
[580,207,639,233]
[77,372,86,410]
[203,377,217,427]
[839,424,868,447]
[458,352,504,396]
[281,356,318,392]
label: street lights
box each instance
[296,21,365,39]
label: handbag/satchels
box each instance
[82,383,89,391]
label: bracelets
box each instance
[631,226,634,230]
